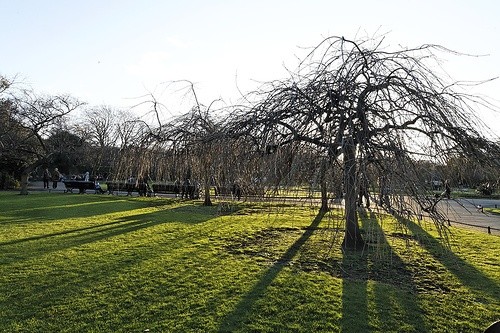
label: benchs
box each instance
[153,184,200,199]
[63,180,98,194]
[106,181,149,197]
[213,185,266,201]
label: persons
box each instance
[42,168,51,189]
[333,173,344,199]
[357,173,370,208]
[434,178,464,191]
[482,181,491,196]
[51,168,60,189]
[60,172,249,201]
[445,179,452,199]
